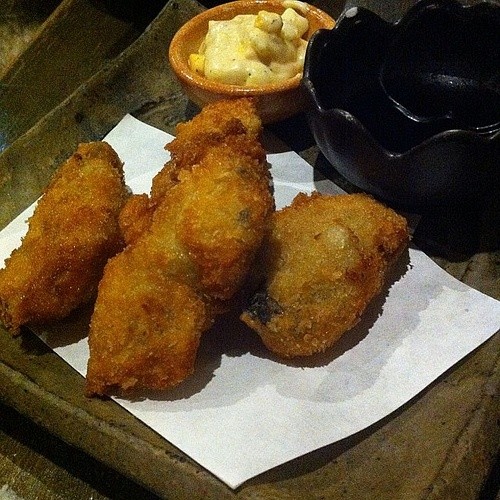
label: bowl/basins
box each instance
[167,0,336,125]
[302,0,500,212]
